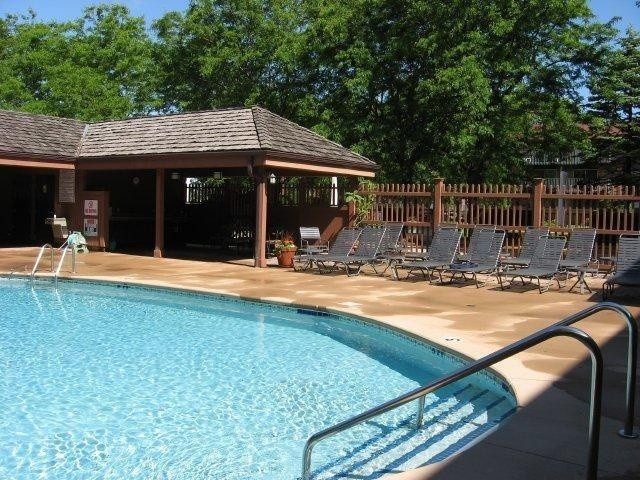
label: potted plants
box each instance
[271,225,298,267]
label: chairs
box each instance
[496,225,639,301]
[300,222,507,290]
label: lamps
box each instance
[168,167,223,180]
[268,171,277,185]
